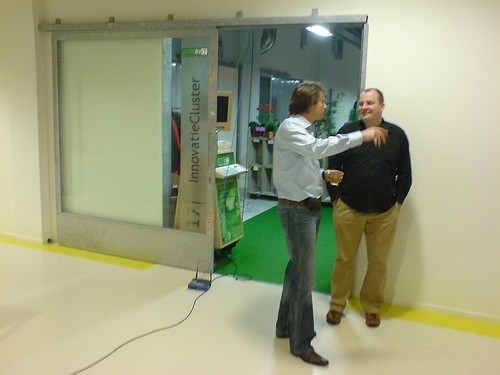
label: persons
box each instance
[272,81,388,366]
[326,89,413,326]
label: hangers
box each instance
[171,106,183,114]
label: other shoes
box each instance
[326,310,342,324]
[297,345,328,365]
[365,312,381,326]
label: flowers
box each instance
[249,104,281,132]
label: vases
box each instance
[250,130,260,137]
[269,131,275,139]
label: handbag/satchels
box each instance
[188,259,213,290]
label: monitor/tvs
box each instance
[215,91,232,131]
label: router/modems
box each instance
[187,267,212,291]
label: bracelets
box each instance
[322,169,326,180]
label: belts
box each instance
[278,196,321,207]
